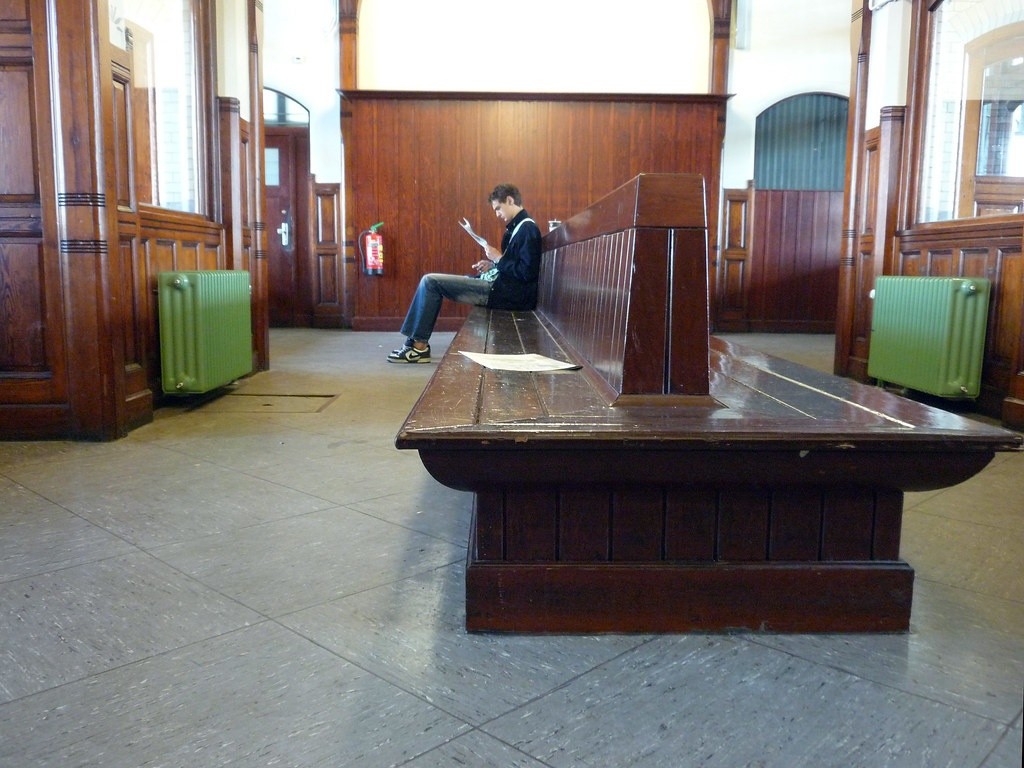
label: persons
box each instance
[387,184,541,363]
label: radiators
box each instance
[866,274,992,394]
[157,270,253,393]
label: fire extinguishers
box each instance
[358,221,385,277]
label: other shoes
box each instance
[387,338,432,364]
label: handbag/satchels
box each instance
[480,268,500,282]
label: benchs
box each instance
[397,172,1023,637]
